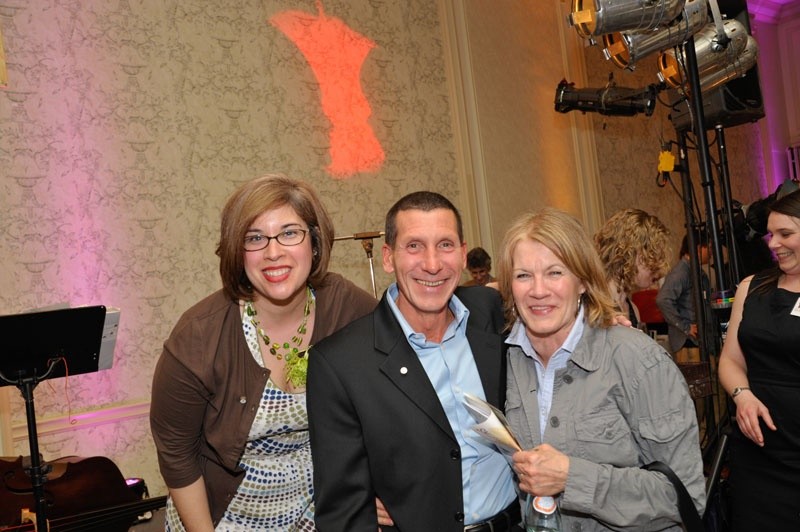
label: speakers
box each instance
[666,0,764,135]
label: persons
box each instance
[456,181,800,532]
[304,189,644,532]
[149,174,380,532]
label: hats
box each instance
[746,179,800,234]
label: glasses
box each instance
[242,229,311,251]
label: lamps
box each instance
[554,72,657,117]
[568,0,757,98]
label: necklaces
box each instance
[245,282,316,389]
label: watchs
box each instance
[731,387,751,399]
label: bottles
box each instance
[525,493,563,532]
